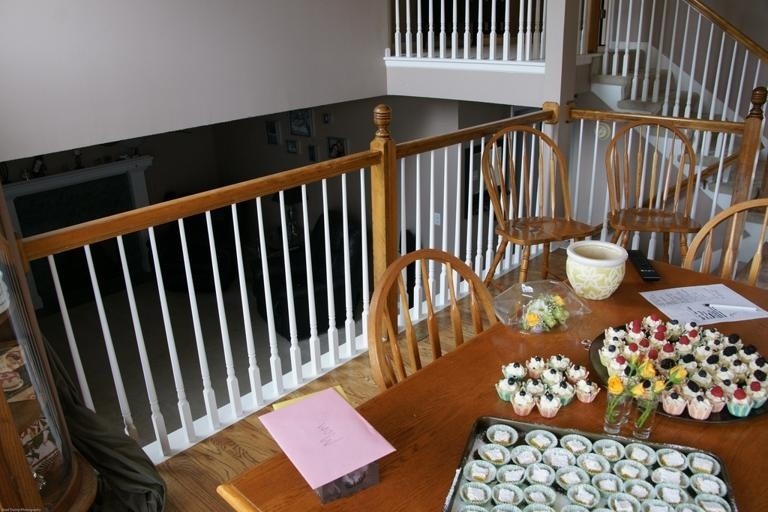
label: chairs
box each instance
[145,191,246,292]
[368,249,500,392]
[368,229,424,308]
[605,119,701,265]
[254,210,365,341]
[483,125,596,318]
[683,198,768,287]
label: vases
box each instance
[633,397,660,440]
[618,376,637,423]
[603,389,629,434]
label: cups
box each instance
[604,375,662,439]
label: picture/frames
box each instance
[465,137,510,219]
[265,108,348,163]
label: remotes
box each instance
[629,250,661,281]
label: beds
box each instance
[235,224,312,296]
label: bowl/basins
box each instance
[565,240,629,301]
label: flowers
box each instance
[607,373,646,424]
[636,364,687,427]
[623,353,657,400]
[520,294,570,332]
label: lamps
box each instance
[272,186,309,245]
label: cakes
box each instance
[495,312,768,420]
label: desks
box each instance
[215,260,768,512]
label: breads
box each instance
[459,423,732,512]
